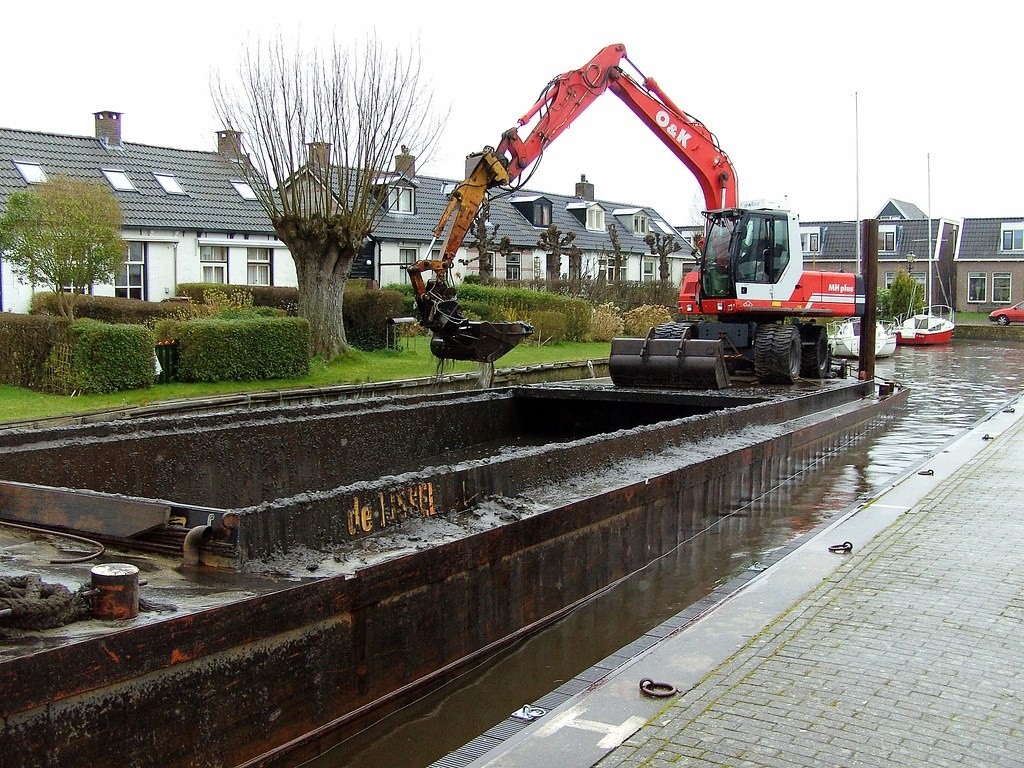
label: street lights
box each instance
[905,250,916,278]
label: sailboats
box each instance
[826,92,898,359]
[889,152,956,346]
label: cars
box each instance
[988,300,1024,327]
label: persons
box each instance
[739,223,768,277]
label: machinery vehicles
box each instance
[407,42,869,390]
